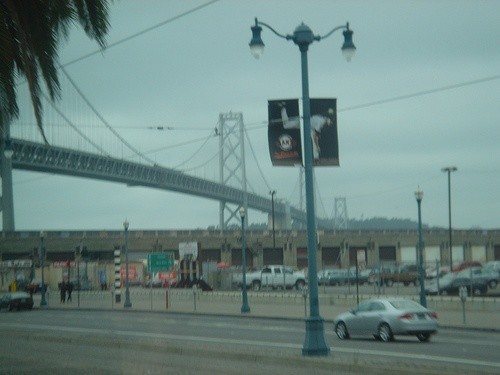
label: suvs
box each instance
[372,262,427,288]
[451,260,483,272]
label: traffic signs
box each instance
[147,251,175,273]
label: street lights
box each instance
[246,16,358,358]
[39,225,49,307]
[238,206,251,313]
[122,218,132,309]
[268,189,276,247]
[440,165,458,272]
[413,184,431,308]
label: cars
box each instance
[333,296,440,343]
[0,284,32,313]
[417,274,489,298]
[457,267,500,289]
[231,263,306,293]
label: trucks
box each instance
[317,268,370,286]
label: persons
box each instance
[58,281,66,303]
[65,281,75,302]
[278,102,333,162]
